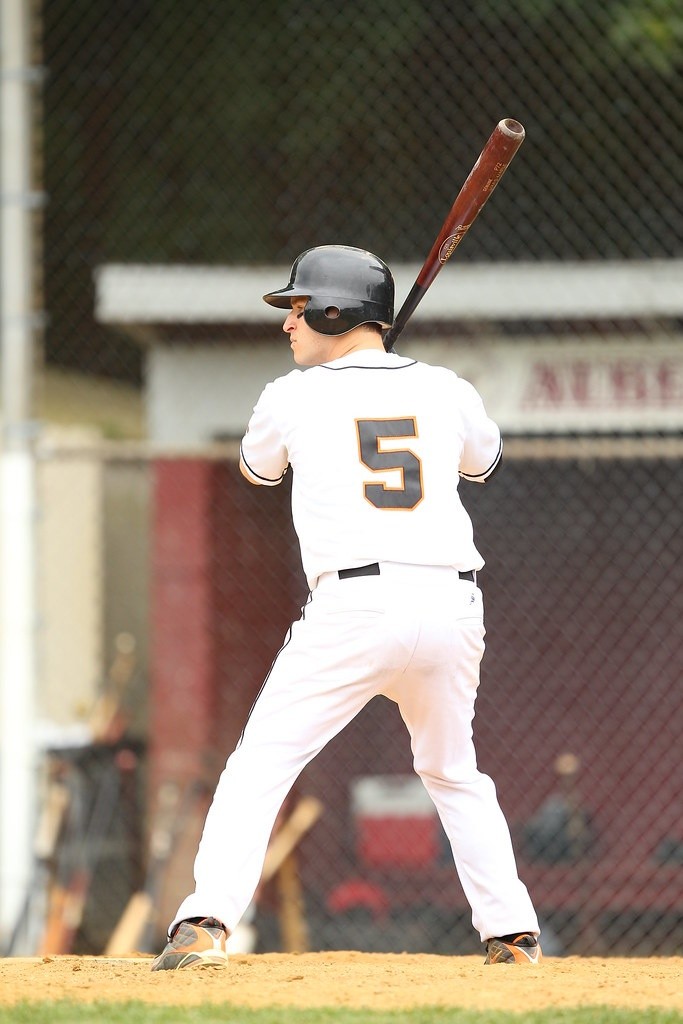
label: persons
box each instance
[152,246,542,972]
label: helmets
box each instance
[262,244,395,336]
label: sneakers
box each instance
[483,935,543,964]
[151,916,228,970]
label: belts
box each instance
[337,562,480,588]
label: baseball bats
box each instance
[384,119,526,352]
